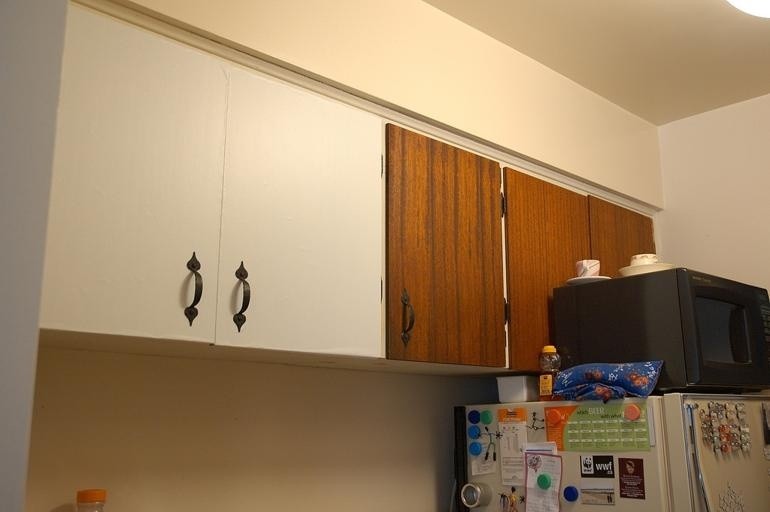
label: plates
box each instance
[565,276,610,284]
[618,263,674,278]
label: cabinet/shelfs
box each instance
[502,166,656,371]
[38,0,386,370]
[386,121,505,369]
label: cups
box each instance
[631,254,658,265]
[575,259,601,276]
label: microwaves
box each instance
[551,267,769,394]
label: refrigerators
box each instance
[453,393,770,512]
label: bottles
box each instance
[74,488,106,512]
[536,345,562,402]
